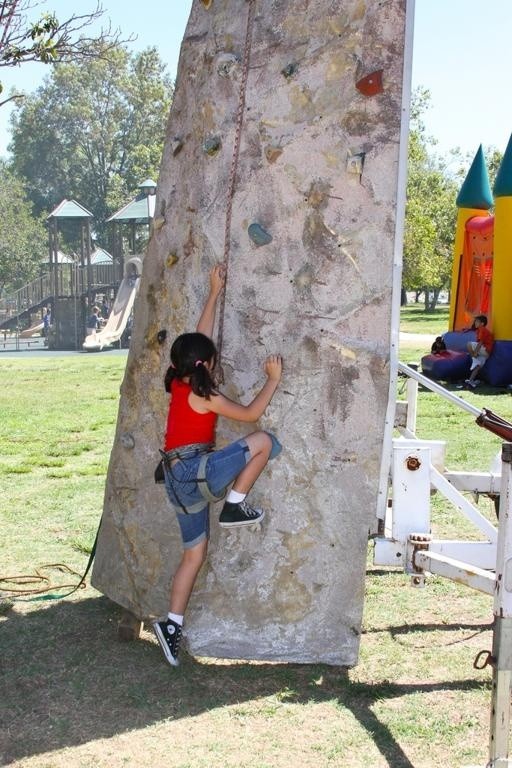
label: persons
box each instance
[152,260,285,668]
[43,307,52,345]
[430,336,446,355]
[465,315,495,386]
[86,305,105,334]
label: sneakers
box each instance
[152,617,182,665]
[470,360,480,370]
[218,500,265,528]
[465,379,477,387]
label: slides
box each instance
[19,322,45,338]
[82,254,143,352]
